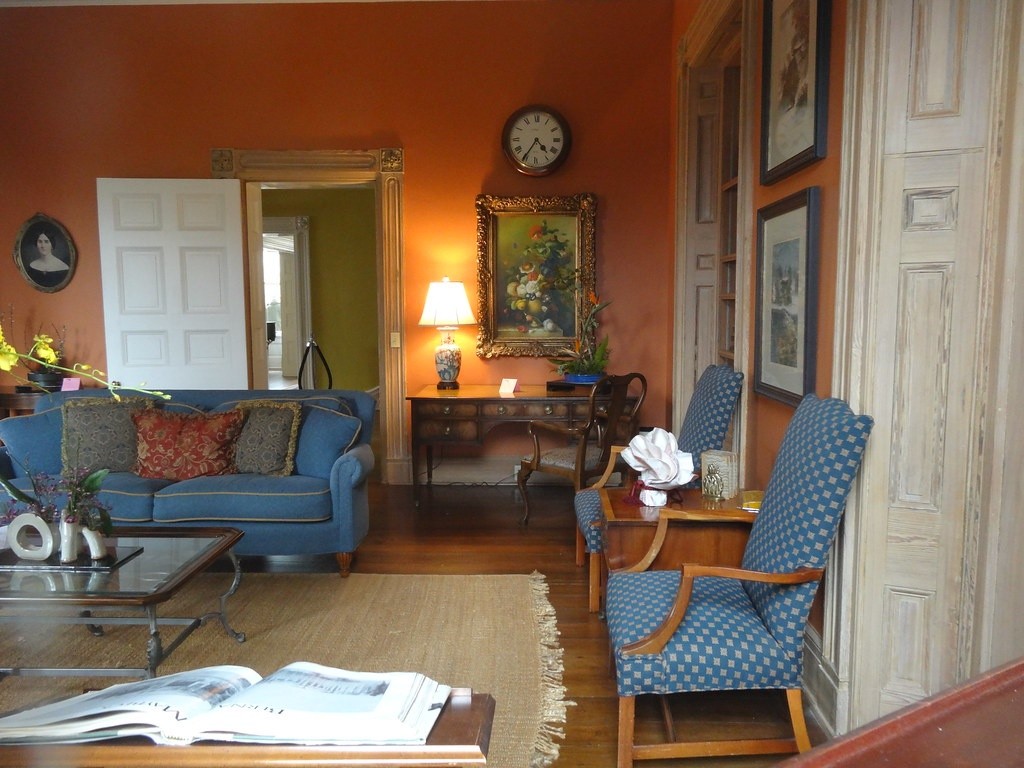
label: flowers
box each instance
[0,305,171,539]
[517,263,613,374]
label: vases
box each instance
[27,373,65,390]
[565,372,608,384]
[0,516,107,563]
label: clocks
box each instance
[501,103,572,177]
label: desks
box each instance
[599,487,756,622]
[0,385,47,417]
[0,688,497,768]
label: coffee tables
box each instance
[0,526,246,681]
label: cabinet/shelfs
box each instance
[405,385,643,507]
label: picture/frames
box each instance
[753,0,833,407]
[474,193,597,358]
[12,212,77,294]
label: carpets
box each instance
[0,573,578,768]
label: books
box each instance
[0,661,452,745]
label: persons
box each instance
[26,231,70,287]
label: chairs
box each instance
[518,364,875,768]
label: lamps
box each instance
[418,276,478,390]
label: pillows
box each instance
[0,397,362,482]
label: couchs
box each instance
[0,388,377,579]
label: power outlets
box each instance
[514,465,521,480]
